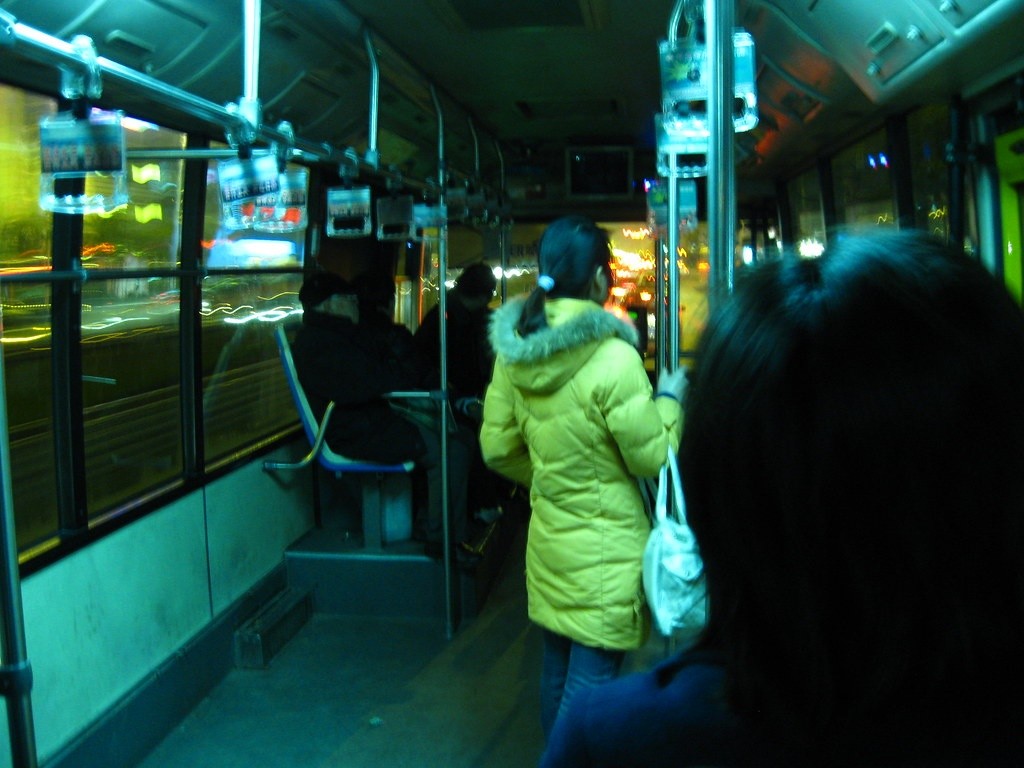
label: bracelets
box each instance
[654,391,678,401]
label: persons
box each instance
[296,217,689,768]
[538,226,1024,768]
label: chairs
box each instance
[272,320,413,554]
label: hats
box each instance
[458,261,497,294]
[299,271,371,310]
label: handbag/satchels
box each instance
[639,444,710,642]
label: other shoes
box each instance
[414,521,487,569]
